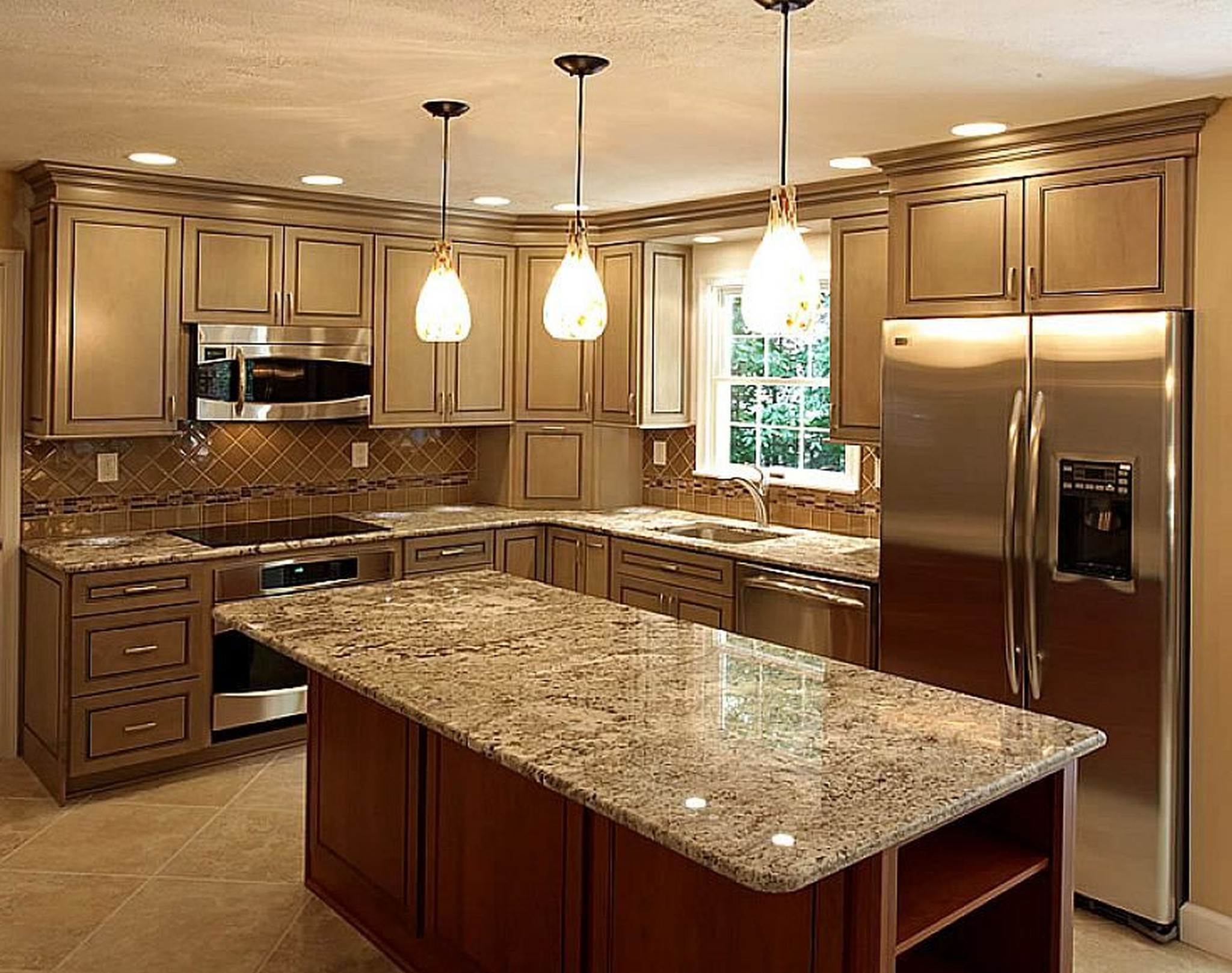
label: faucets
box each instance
[717,466,771,525]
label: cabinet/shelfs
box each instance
[369,232,516,426]
[831,212,888,449]
[179,216,374,328]
[514,245,595,426]
[402,534,494,576]
[591,243,697,431]
[888,155,1197,315]
[545,525,610,598]
[208,567,1107,973]
[494,526,545,585]
[609,536,736,628]
[21,559,213,780]
[24,205,181,438]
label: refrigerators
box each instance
[879,308,1192,946]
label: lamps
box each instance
[739,1,822,333]
[541,54,608,342]
[414,100,473,344]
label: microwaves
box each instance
[189,325,374,422]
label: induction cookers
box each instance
[165,515,392,550]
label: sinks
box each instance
[646,520,795,545]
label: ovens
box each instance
[210,550,394,744]
